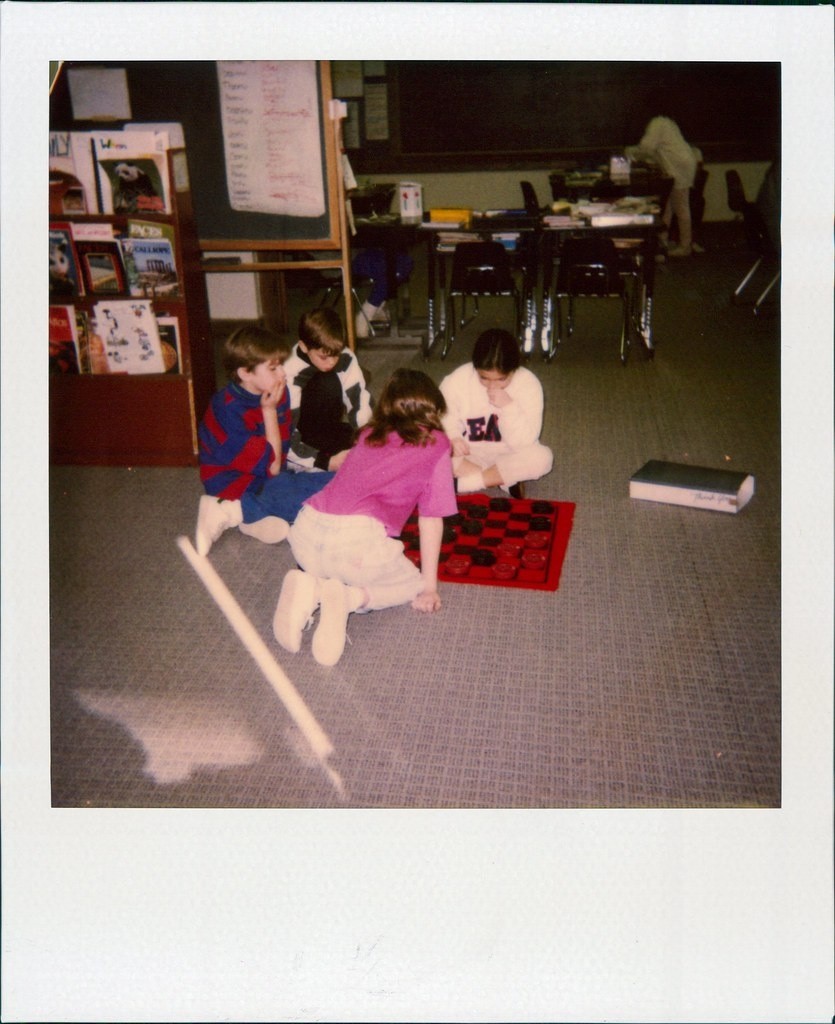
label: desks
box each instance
[549,172,671,213]
[354,207,666,346]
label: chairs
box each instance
[309,168,781,365]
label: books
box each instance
[436,232,480,252]
[628,459,755,514]
[49,133,183,375]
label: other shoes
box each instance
[273,569,320,653]
[196,494,230,559]
[510,482,526,500]
[668,240,693,257]
[356,335,381,351]
[238,516,290,545]
[311,579,358,666]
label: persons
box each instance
[282,308,375,473]
[624,103,696,255]
[311,188,414,339]
[437,328,553,493]
[195,325,336,558]
[272,367,457,666]
